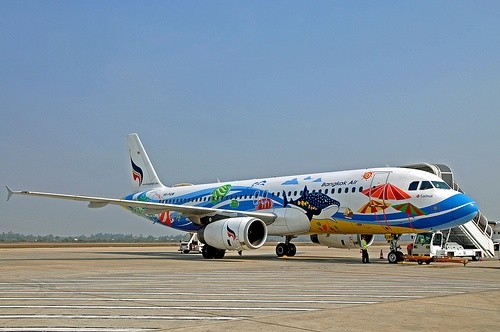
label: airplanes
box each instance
[6,134,478,259]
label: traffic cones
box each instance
[379,250,384,259]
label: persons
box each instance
[360,236,372,263]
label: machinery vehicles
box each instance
[411,231,494,261]
